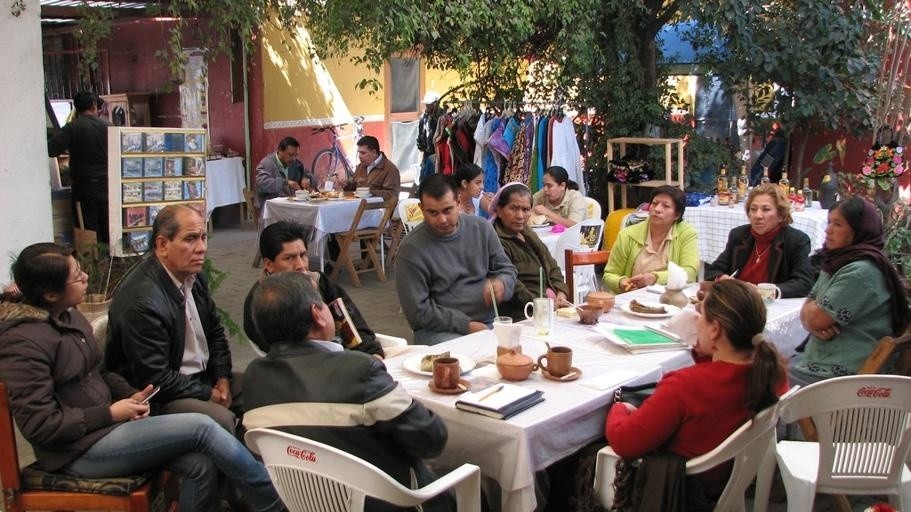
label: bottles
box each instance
[713,164,812,212]
[819,162,838,209]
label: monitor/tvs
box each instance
[46,98,75,130]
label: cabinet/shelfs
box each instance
[96,89,155,127]
[106,126,211,266]
[606,137,688,217]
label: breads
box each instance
[328,190,345,198]
[528,214,547,226]
[421,349,452,373]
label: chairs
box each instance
[367,178,422,267]
[240,425,485,511]
[774,316,911,511]
[0,374,177,510]
[244,319,411,355]
[591,373,801,511]
[241,184,273,271]
[779,369,911,510]
[326,192,398,287]
[532,188,621,303]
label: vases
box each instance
[816,161,846,212]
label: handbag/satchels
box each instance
[613,383,657,408]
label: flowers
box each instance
[860,139,911,186]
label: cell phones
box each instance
[137,386,160,405]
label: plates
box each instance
[312,196,324,201]
[291,197,307,202]
[327,196,343,202]
[354,195,373,198]
[619,297,679,320]
[530,223,551,228]
[402,350,477,377]
[428,378,470,395]
[541,366,583,382]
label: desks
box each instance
[628,187,842,275]
[206,157,247,234]
[360,277,829,512]
[261,188,391,277]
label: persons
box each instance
[324,135,400,274]
[563,279,789,512]
[47,89,115,241]
[255,138,311,202]
[602,184,700,297]
[245,221,429,361]
[704,182,814,299]
[454,161,494,220]
[533,167,588,230]
[489,181,568,325]
[395,173,518,348]
[776,198,911,440]
[1,241,289,512]
[240,270,490,512]
[103,206,248,512]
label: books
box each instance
[455,383,545,419]
[328,298,363,350]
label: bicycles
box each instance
[309,117,369,196]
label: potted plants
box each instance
[66,251,126,325]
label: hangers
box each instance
[431,101,572,124]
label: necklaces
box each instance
[756,241,771,263]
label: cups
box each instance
[495,321,523,348]
[758,282,782,307]
[532,297,554,337]
[295,190,310,199]
[496,350,539,382]
[325,182,335,191]
[698,280,717,300]
[493,314,511,327]
[536,346,573,376]
[355,187,369,195]
[586,292,616,313]
[524,301,535,325]
[575,305,605,325]
[430,358,460,390]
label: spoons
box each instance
[460,361,490,375]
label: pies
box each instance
[630,299,666,314]
